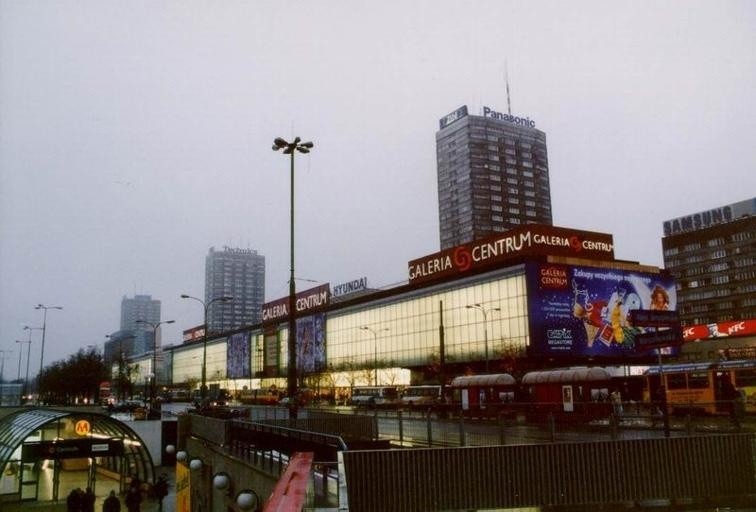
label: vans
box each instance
[172,391,187,402]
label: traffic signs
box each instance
[634,327,684,351]
[626,310,681,327]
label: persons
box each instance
[80,486,96,512]
[103,488,121,512]
[123,486,142,511]
[67,488,81,511]
[128,472,141,489]
[650,286,674,356]
[156,476,169,510]
[611,389,627,420]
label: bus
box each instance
[240,390,279,405]
[402,385,453,406]
[352,387,400,410]
[193,389,219,401]
[643,361,756,415]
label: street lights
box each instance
[365,327,389,387]
[272,137,313,428]
[16,341,32,380]
[23,326,44,388]
[466,304,500,370]
[0,351,12,380]
[181,295,233,398]
[106,335,138,403]
[136,320,176,387]
[35,304,63,376]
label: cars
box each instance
[279,398,289,407]
